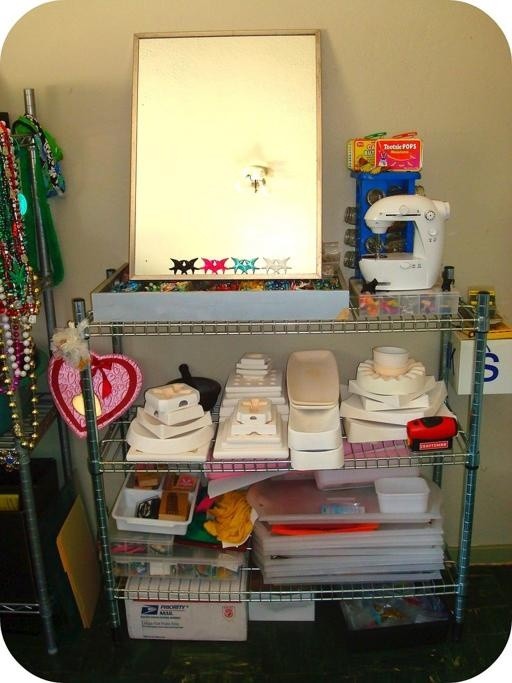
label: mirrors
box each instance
[129,28,323,280]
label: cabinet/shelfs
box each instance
[71,264,491,627]
[0,88,75,657]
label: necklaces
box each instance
[0,124,43,473]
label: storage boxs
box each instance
[124,560,247,642]
[90,261,350,321]
